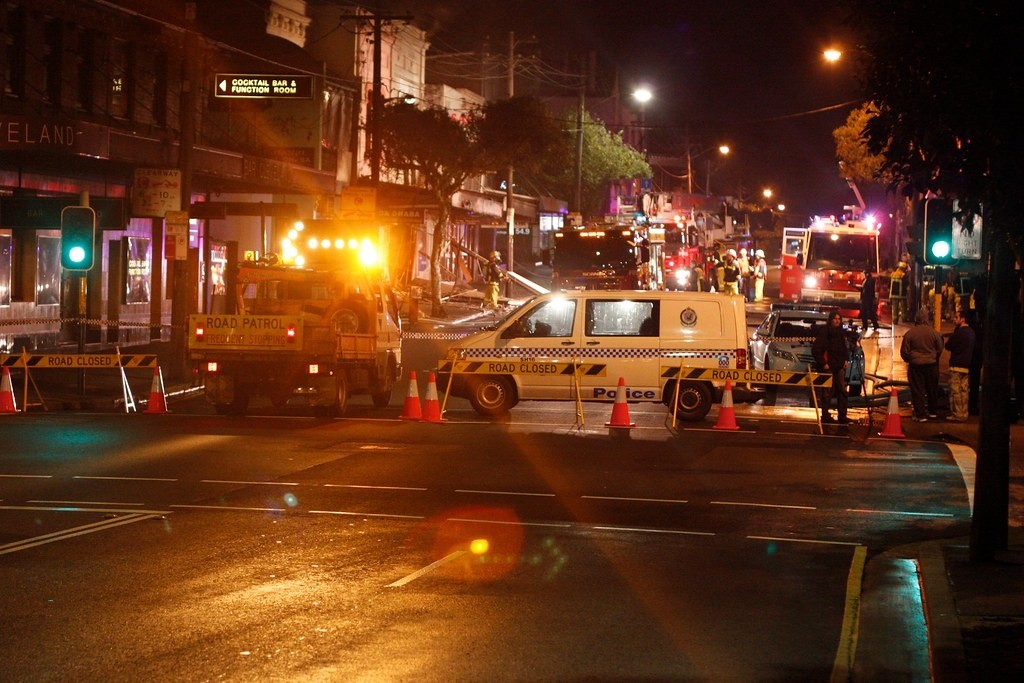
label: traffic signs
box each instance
[215,71,315,99]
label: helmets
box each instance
[727,249,736,258]
[739,248,748,254]
[755,250,765,258]
[489,251,501,258]
[898,261,906,269]
[901,253,910,260]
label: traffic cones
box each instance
[420,371,448,423]
[0,365,22,414]
[877,390,906,437]
[605,376,639,428]
[399,369,423,421]
[141,367,174,413]
[713,380,739,430]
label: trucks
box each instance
[188,260,419,415]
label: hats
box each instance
[915,308,928,322]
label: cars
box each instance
[749,309,865,395]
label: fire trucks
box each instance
[541,220,667,292]
[780,158,882,311]
[636,193,754,293]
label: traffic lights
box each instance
[907,197,960,266]
[61,207,93,271]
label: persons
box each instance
[483,251,506,308]
[889,254,984,422]
[812,309,855,424]
[127,277,147,302]
[691,248,767,302]
[848,271,881,331]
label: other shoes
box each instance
[859,325,868,330]
[946,415,967,423]
[821,416,839,424]
[912,415,928,422]
[838,416,855,424]
[873,325,879,331]
[927,410,937,418]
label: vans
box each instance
[434,288,766,424]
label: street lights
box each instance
[687,144,729,194]
[574,87,653,210]
[738,189,771,234]
